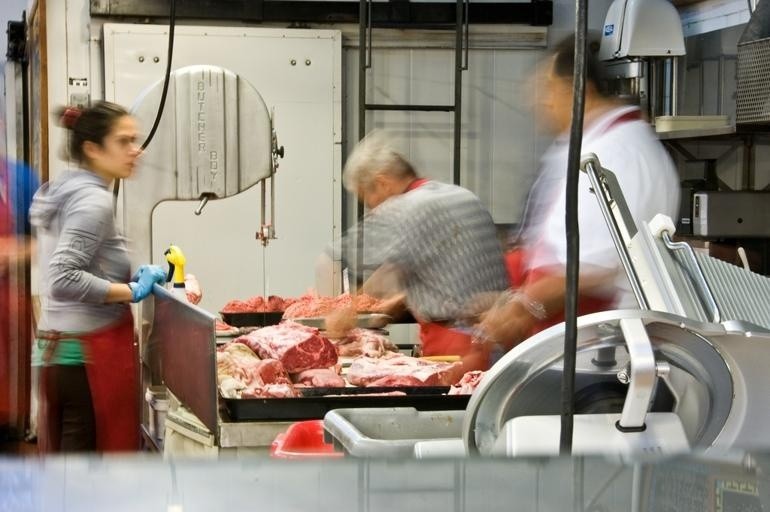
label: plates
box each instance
[292,312,391,329]
[219,307,284,328]
[214,386,472,423]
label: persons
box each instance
[29,100,168,459]
[0,157,41,460]
[312,128,522,374]
[452,30,681,347]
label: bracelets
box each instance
[514,291,552,325]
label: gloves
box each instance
[129,267,167,304]
[132,265,155,282]
